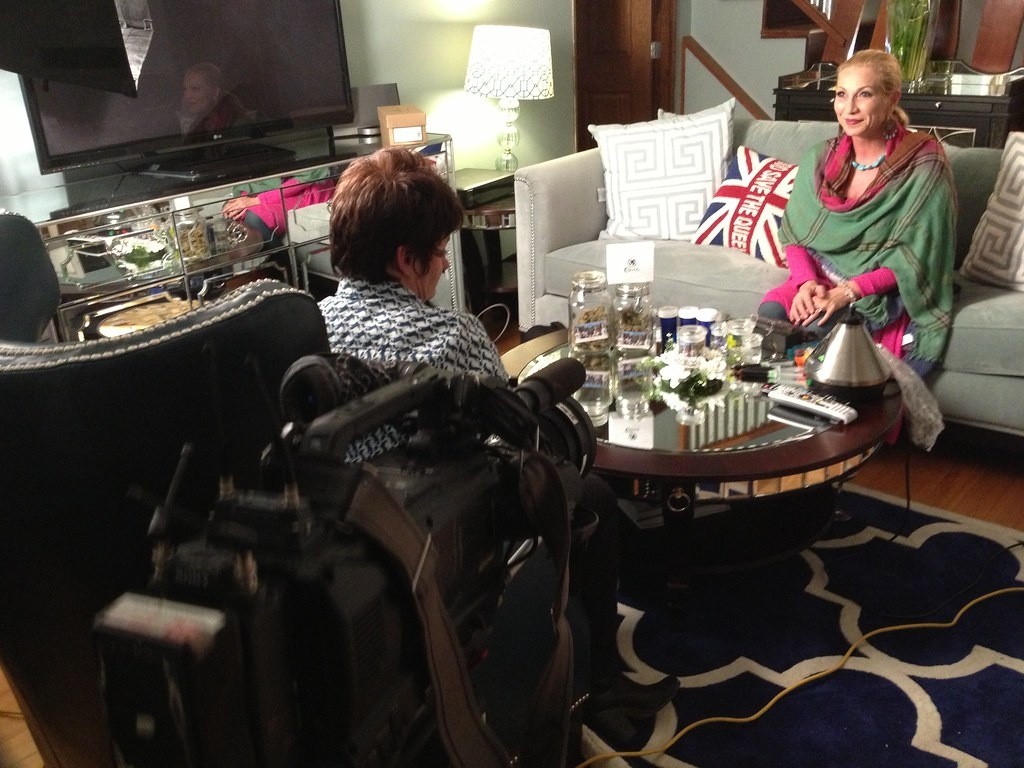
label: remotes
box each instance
[761,383,859,425]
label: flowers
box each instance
[634,333,746,426]
[116,236,167,273]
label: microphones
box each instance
[512,357,586,411]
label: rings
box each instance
[816,307,825,316]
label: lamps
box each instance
[463,24,555,172]
[350,83,400,136]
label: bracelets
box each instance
[838,279,859,302]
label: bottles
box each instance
[562,266,767,431]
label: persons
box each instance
[318,146,680,745]
[758,50,959,452]
[182,63,336,281]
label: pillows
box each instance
[587,97,799,269]
[957,131,1024,293]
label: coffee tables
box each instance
[501,328,903,618]
[97,300,210,338]
[461,196,518,293]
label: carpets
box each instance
[580,486,1024,768]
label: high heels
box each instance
[584,674,680,748]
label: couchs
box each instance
[0,214,337,768]
[513,120,1024,438]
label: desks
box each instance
[772,62,1024,149]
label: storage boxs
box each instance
[377,105,426,148]
[456,169,514,210]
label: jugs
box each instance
[808,301,891,395]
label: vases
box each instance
[884,0,940,91]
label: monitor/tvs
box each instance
[17,0,355,175]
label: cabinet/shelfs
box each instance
[0,132,463,345]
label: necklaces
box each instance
[851,153,886,171]
